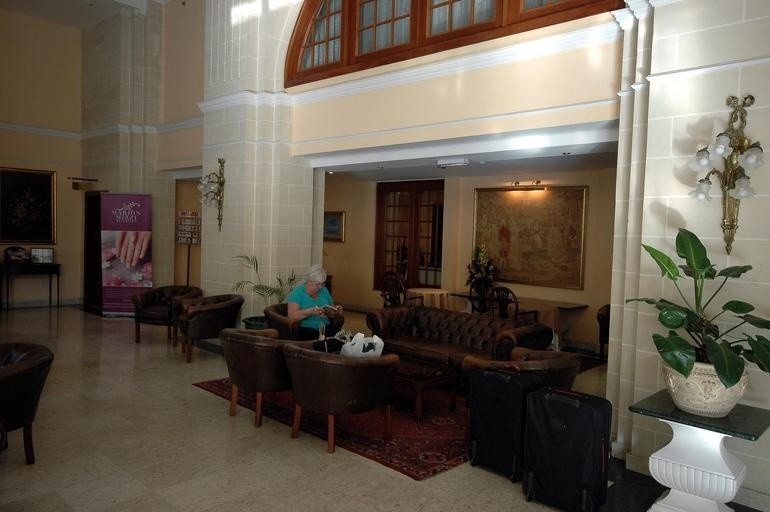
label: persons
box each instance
[287,265,343,340]
[115,230,151,269]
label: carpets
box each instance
[190,353,509,484]
[559,343,608,379]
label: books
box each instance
[320,304,342,315]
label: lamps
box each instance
[690,91,762,255]
[508,174,548,193]
[196,154,227,232]
[177,208,203,288]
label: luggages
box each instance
[523,387,612,512]
[467,366,542,483]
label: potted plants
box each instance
[628,227,770,418]
[230,250,307,330]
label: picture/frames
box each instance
[324,211,346,244]
[471,186,590,290]
[30,248,55,263]
[1,167,59,246]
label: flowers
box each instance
[466,239,492,287]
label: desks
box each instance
[405,285,450,312]
[1,263,64,314]
[451,289,588,323]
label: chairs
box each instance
[379,274,425,307]
[488,284,538,325]
[467,281,494,319]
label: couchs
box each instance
[462,342,584,480]
[0,340,53,464]
[266,300,345,340]
[368,304,554,397]
[179,292,245,362]
[596,304,610,351]
[286,336,400,455]
[218,324,312,428]
[133,284,202,344]
